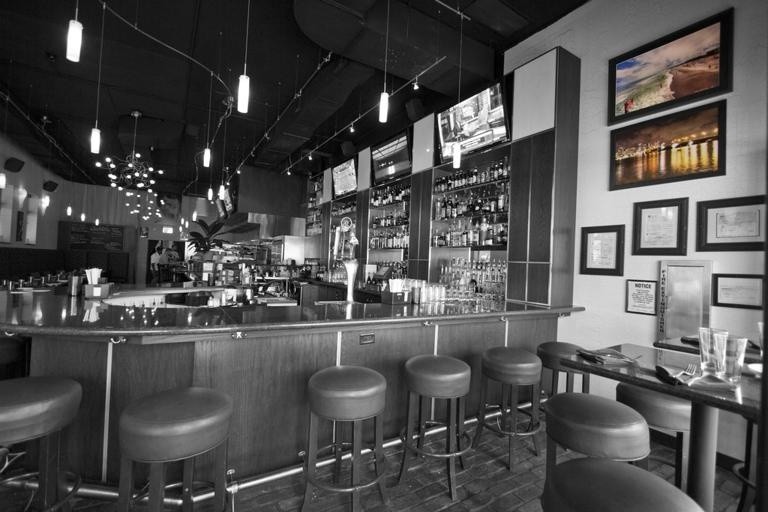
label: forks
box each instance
[669,362,696,381]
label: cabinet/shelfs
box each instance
[304,46,581,308]
[56,221,129,283]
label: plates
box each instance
[16,288,32,292]
[56,280,69,282]
[747,363,763,378]
[46,283,61,286]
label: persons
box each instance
[158,247,174,282]
[149,246,163,284]
[171,243,180,280]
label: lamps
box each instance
[207,152,213,201]
[378,0,391,123]
[237,0,251,114]
[94,111,165,191]
[281,55,448,176]
[65,0,83,63]
[452,13,463,169]
[192,181,198,222]
[219,118,227,201]
[90,1,106,154]
[202,72,214,168]
[124,188,166,222]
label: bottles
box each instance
[371,184,410,208]
[364,272,382,286]
[441,255,506,272]
[434,182,509,220]
[333,201,356,216]
[432,214,507,245]
[372,211,409,228]
[370,227,408,248]
[434,156,511,193]
[374,261,407,277]
[439,273,504,295]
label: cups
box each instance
[726,336,747,381]
[68,271,83,296]
[711,329,728,375]
[699,327,711,371]
